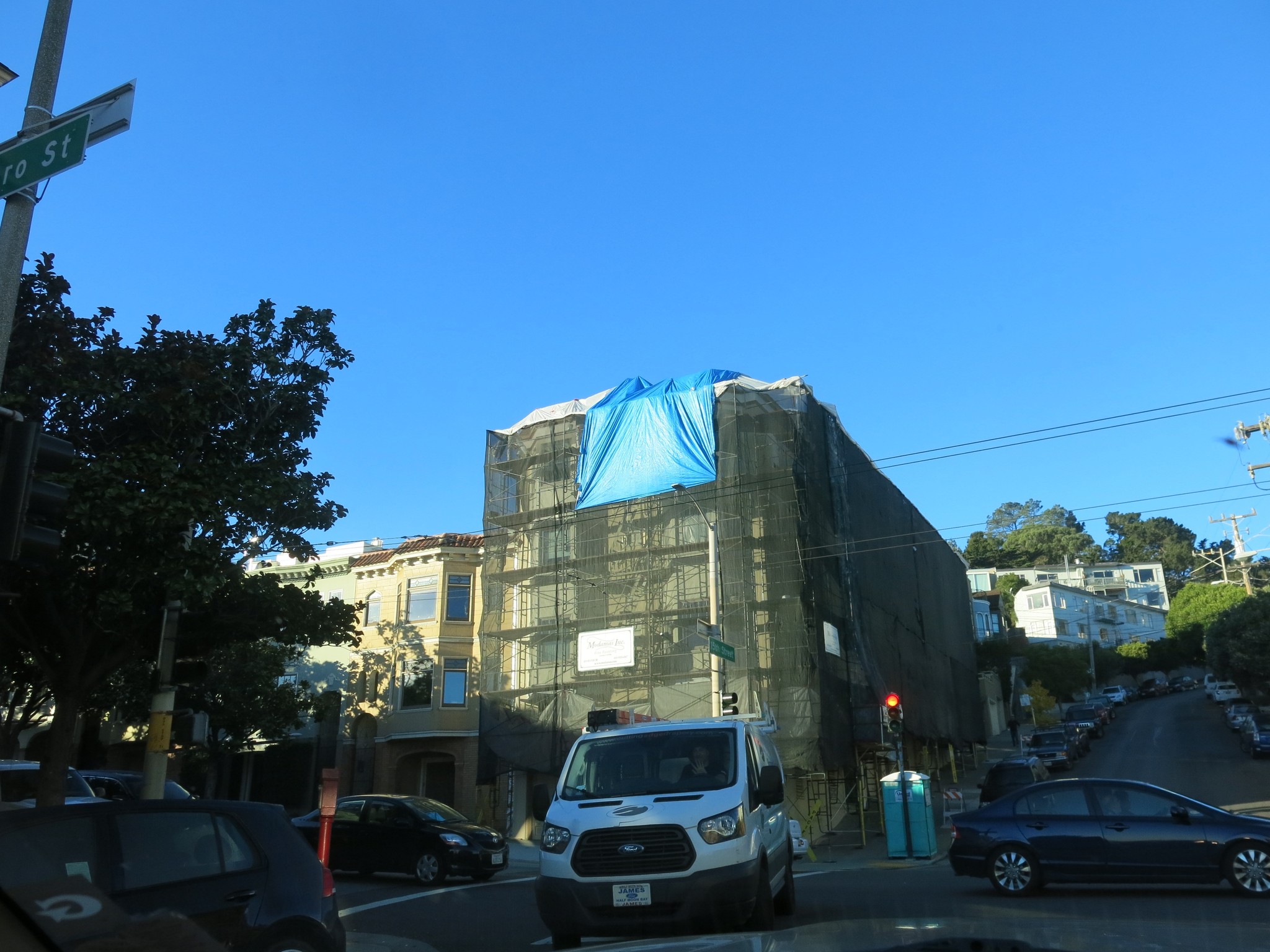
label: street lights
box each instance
[671,482,725,717]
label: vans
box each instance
[531,717,796,950]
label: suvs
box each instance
[1026,702,1105,771]
[1099,685,1128,707]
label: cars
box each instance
[1168,675,1199,694]
[1122,685,1135,702]
[292,794,510,889]
[976,755,1050,808]
[1086,695,1116,720]
[1137,676,1171,698]
[0,757,349,951]
[946,776,1270,900]
[1202,673,1270,760]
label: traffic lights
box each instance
[719,689,739,722]
[884,692,904,733]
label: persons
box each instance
[1102,795,1122,816]
[679,743,725,782]
[1113,790,1133,815]
[1007,714,1020,748]
